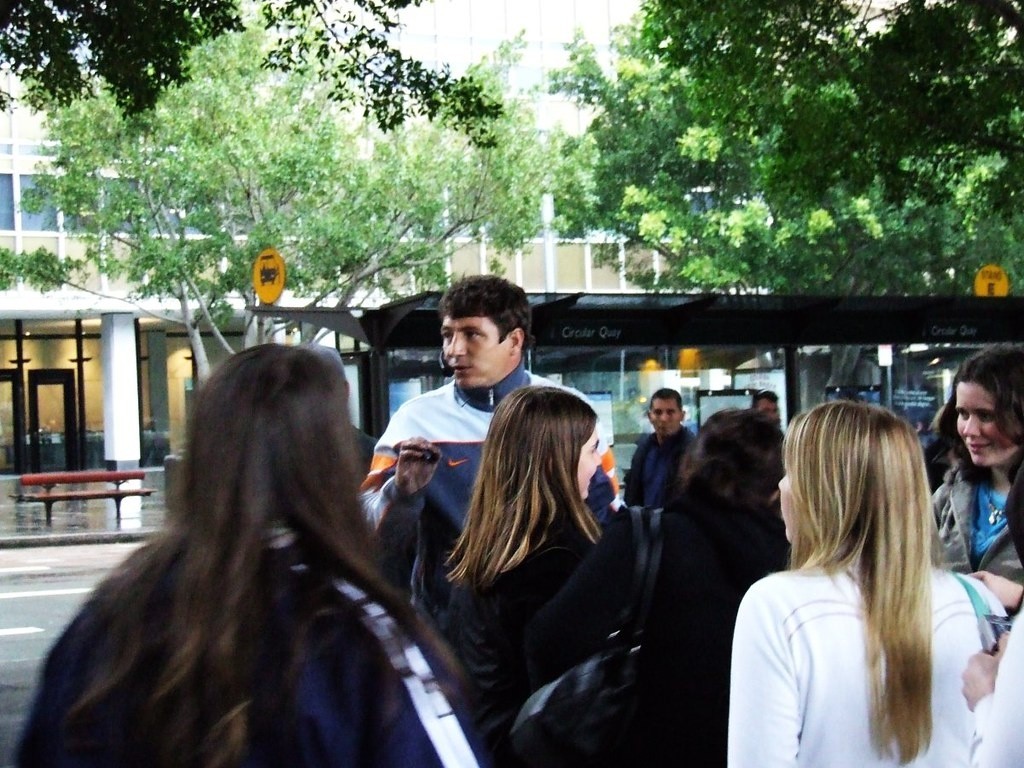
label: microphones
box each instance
[440,351,455,377]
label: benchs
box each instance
[8,471,158,524]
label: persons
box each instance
[359,276,624,635]
[359,342,1024,768]
[18,344,494,768]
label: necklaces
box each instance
[987,496,1003,527]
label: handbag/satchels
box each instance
[506,508,685,768]
